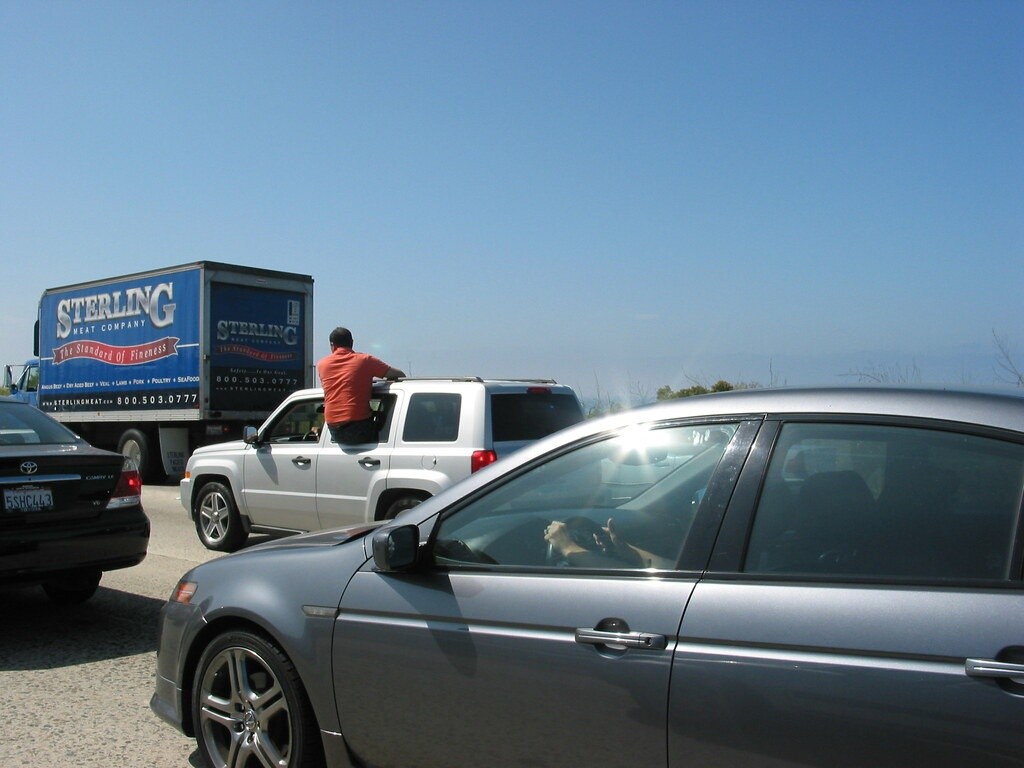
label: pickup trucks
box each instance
[177,374,693,553]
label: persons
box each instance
[318,327,407,443]
[541,517,679,570]
[312,413,327,444]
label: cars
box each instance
[147,386,1023,768]
[0,392,151,628]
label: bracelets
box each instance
[315,433,319,436]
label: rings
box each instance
[545,528,549,533]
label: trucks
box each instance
[2,261,318,486]
